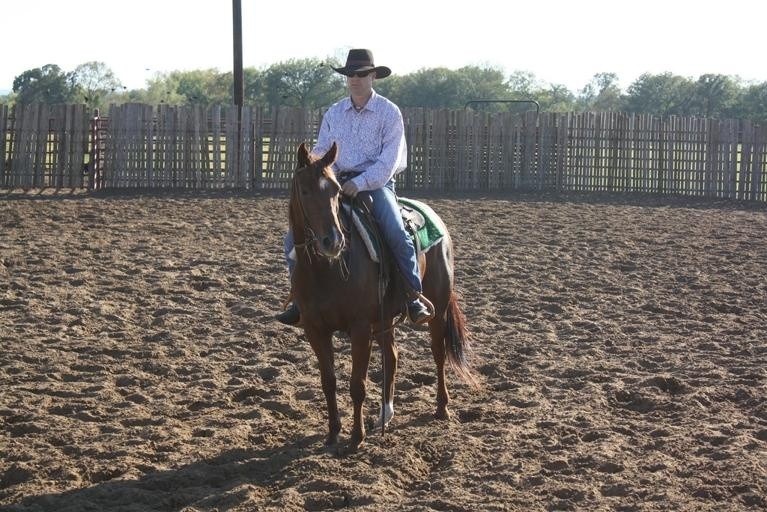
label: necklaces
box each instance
[354,104,363,111]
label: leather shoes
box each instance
[277,308,299,324]
[408,302,430,322]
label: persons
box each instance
[275,48,430,327]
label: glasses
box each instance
[347,71,373,77]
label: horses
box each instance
[287,140,482,456]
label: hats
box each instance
[330,49,391,78]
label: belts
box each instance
[336,171,357,179]
